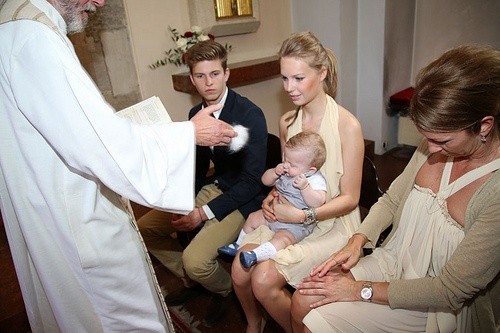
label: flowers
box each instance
[149,26,233,71]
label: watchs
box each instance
[360,281,373,303]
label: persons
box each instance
[217,131,327,269]
[291,44,500,333]
[135,39,267,325]
[0,0,238,333]
[231,32,365,333]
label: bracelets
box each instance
[198,207,205,223]
[303,208,316,226]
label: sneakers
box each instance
[203,291,236,328]
[164,285,199,306]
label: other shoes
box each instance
[240,249,257,268]
[217,242,238,256]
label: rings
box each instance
[332,258,337,264]
